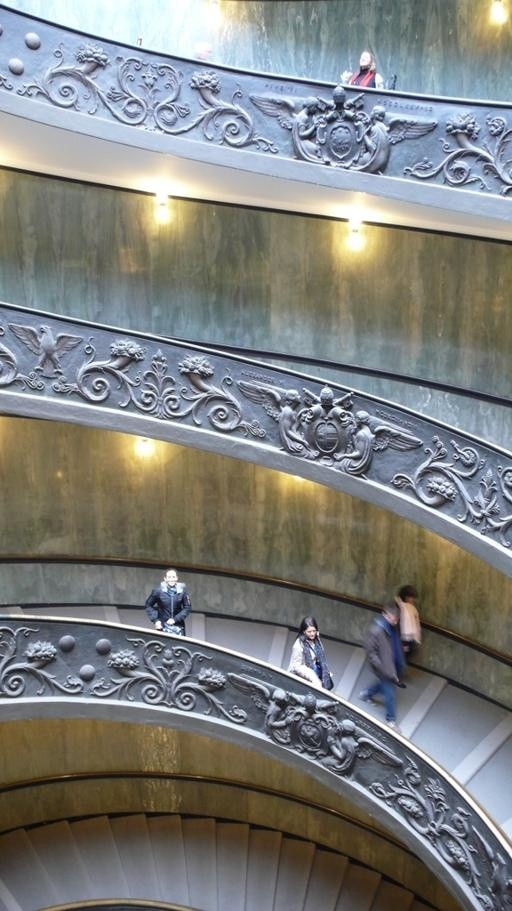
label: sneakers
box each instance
[359,690,379,706]
[384,720,402,735]
[393,678,406,688]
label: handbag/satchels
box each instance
[163,622,185,636]
[320,662,334,690]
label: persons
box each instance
[333,409,374,476]
[144,566,192,637]
[319,719,357,778]
[351,106,392,174]
[291,95,326,165]
[357,602,406,732]
[279,390,310,455]
[393,583,423,688]
[262,689,294,742]
[339,49,386,91]
[287,615,332,695]
